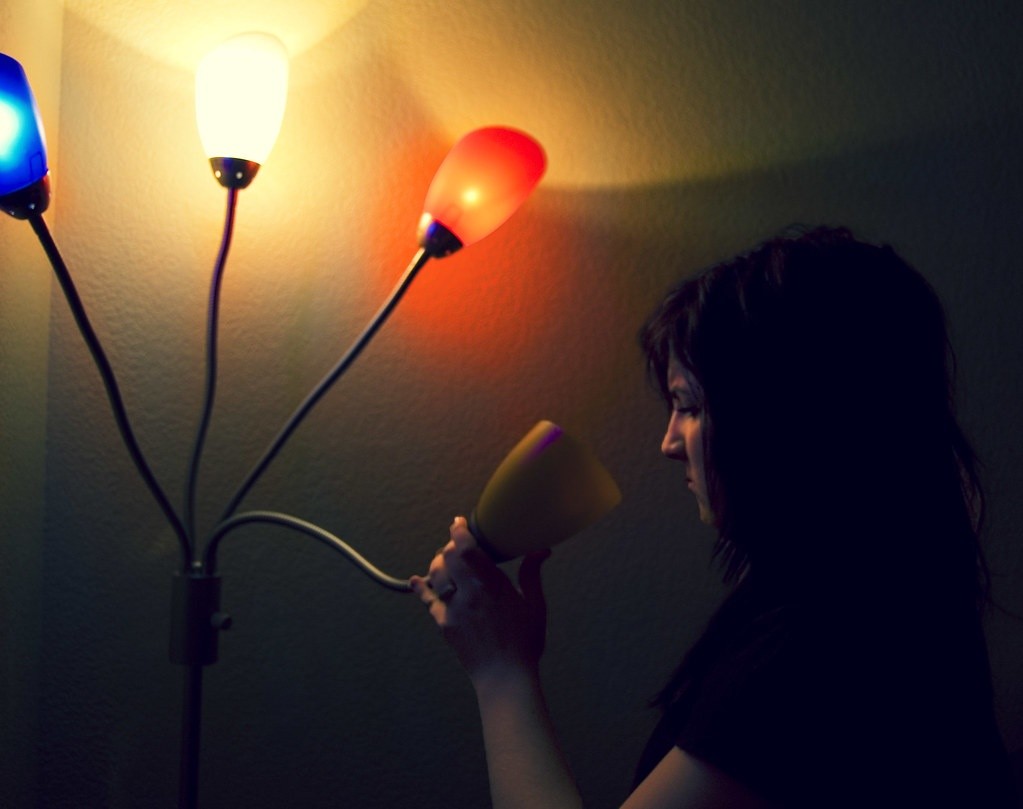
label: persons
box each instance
[409,224,1006,809]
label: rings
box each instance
[439,585,455,601]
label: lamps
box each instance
[1,33,622,809]
